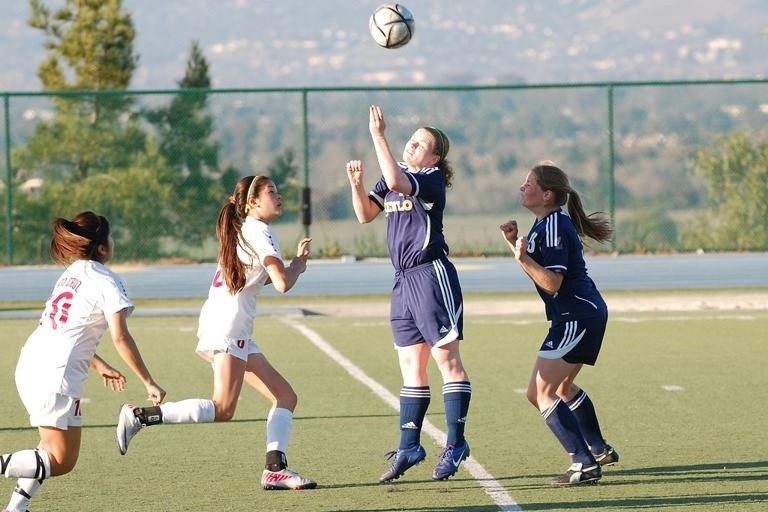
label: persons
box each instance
[0,209,167,512]
[115,172,319,491]
[342,103,474,485]
[497,162,620,487]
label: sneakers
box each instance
[115,403,150,455]
[588,444,619,467]
[379,443,426,484]
[261,467,317,490]
[432,435,470,480]
[550,461,602,487]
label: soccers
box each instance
[368,3,415,49]
